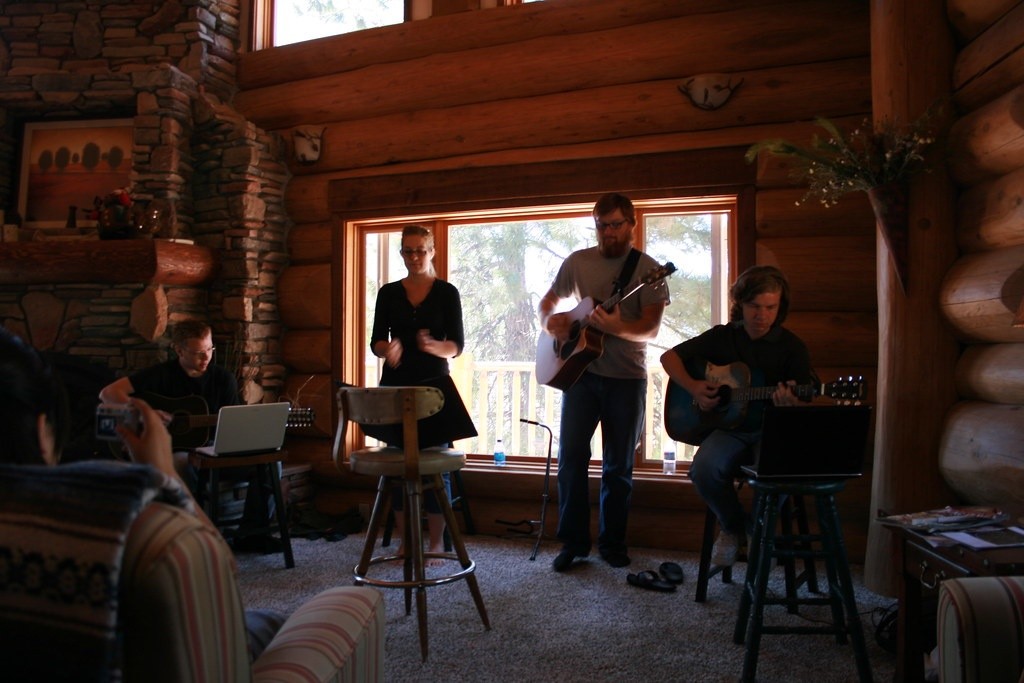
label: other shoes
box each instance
[233,527,286,554]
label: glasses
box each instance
[595,218,627,231]
[400,246,430,256]
[180,343,216,358]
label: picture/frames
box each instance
[18,116,134,230]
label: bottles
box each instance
[494,439,506,467]
[662,438,677,475]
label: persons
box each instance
[370,226,464,567]
[100,319,291,554]
[660,265,819,567]
[540,192,671,573]
[0,396,287,665]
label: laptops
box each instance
[195,402,291,458]
[740,405,871,480]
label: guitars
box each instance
[664,363,869,446]
[110,391,317,463]
[535,261,679,393]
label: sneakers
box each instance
[745,528,778,572]
[710,530,739,566]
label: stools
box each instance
[728,462,873,683]
[691,437,825,613]
[190,447,294,574]
[327,383,498,660]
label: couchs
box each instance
[935,571,1024,683]
[0,456,386,683]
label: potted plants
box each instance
[742,97,951,294]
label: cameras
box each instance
[96,404,137,441]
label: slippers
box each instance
[659,562,683,585]
[627,571,677,593]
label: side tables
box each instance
[880,504,1024,683]
